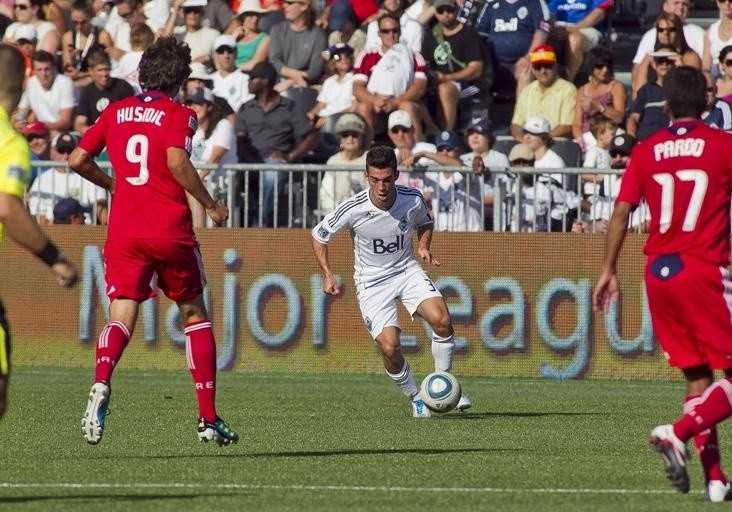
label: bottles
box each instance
[334,110,552,162]
[530,44,556,65]
[608,134,637,152]
[179,0,279,105]
[23,120,75,154]
[329,42,354,58]
[431,0,460,12]
[17,24,35,40]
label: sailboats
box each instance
[420,371,462,413]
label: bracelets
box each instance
[601,106,605,112]
[289,154,294,161]
[34,235,66,268]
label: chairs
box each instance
[649,423,692,494]
[81,380,110,445]
[706,476,731,503]
[456,392,469,411]
[412,394,434,418]
[196,412,240,445]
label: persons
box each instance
[0,0,731,236]
[0,44,82,422]
[69,35,239,445]
[590,65,731,502]
[310,145,470,421]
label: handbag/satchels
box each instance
[34,235,66,268]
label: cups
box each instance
[12,3,134,25]
[657,26,677,33]
[380,27,399,35]
[610,152,629,157]
[533,63,553,69]
[723,58,732,65]
